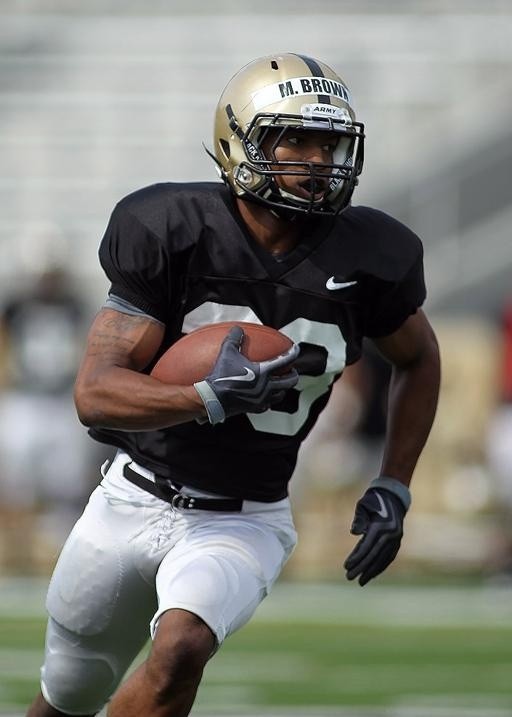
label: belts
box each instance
[122,464,243,513]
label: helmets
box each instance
[213,51,365,222]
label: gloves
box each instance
[192,324,300,425]
[344,477,411,587]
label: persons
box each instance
[3,242,103,573]
[29,48,445,716]
[290,300,512,586]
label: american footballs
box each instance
[150,321,295,386]
